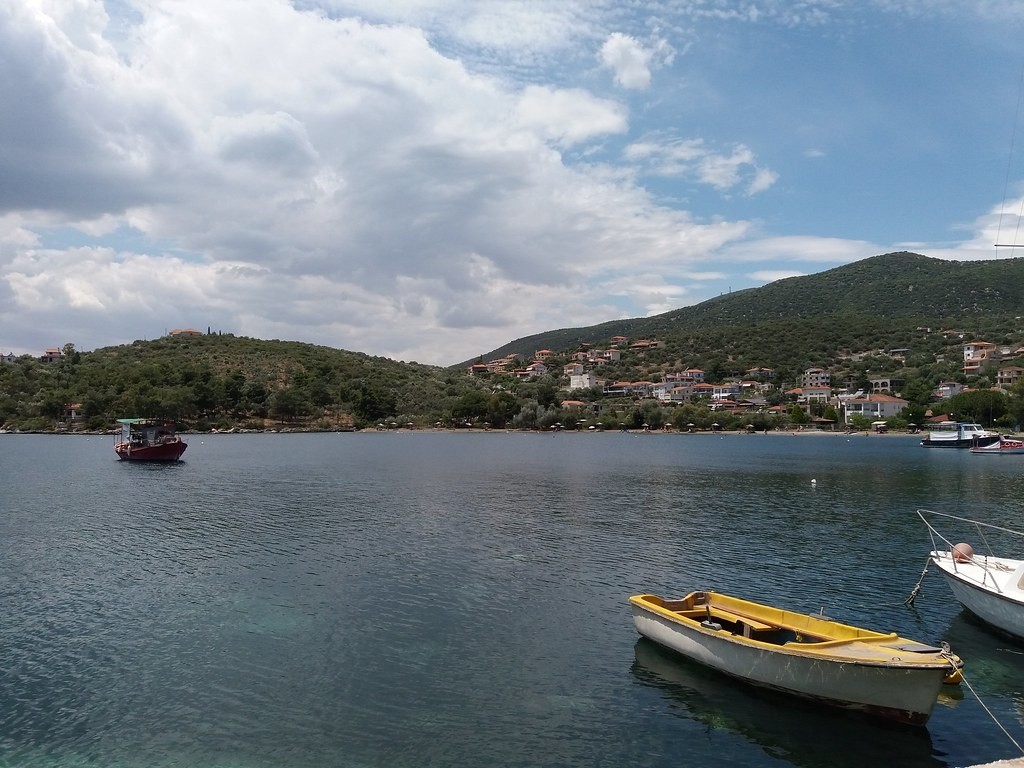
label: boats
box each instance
[630,589,963,729]
[112,416,188,463]
[917,506,1024,641]
[920,422,1011,448]
[970,439,1024,454]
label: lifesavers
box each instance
[1005,442,1011,447]
[1012,442,1017,447]
